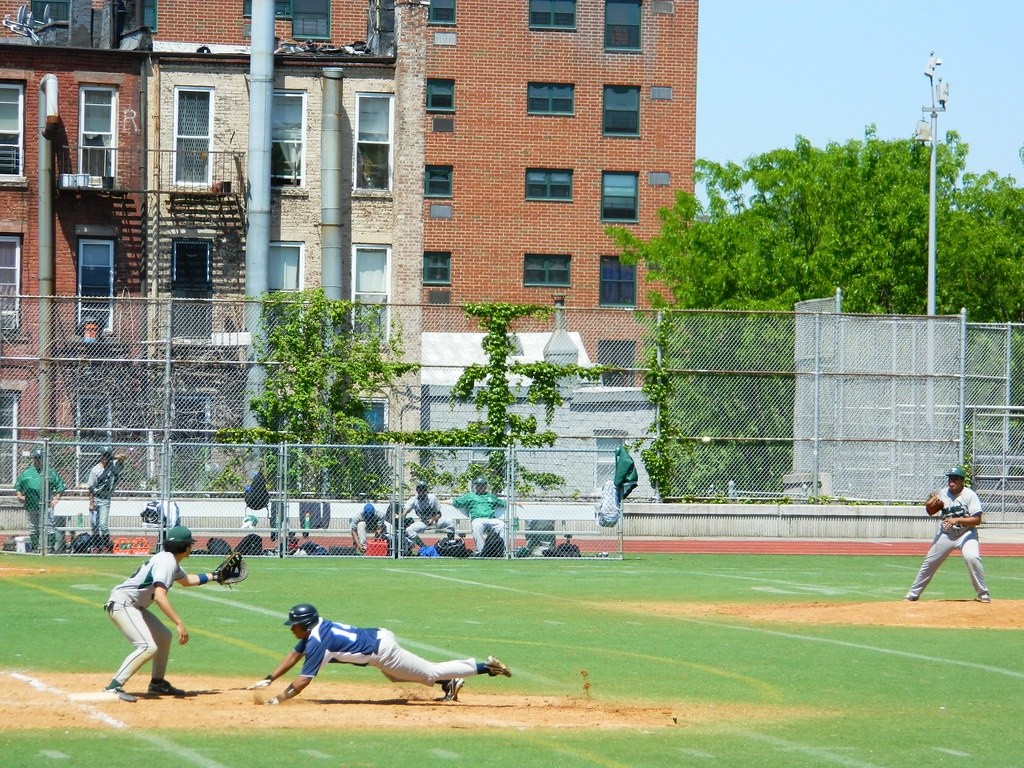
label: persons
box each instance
[15,450,68,554]
[247,602,512,705]
[901,467,990,603]
[438,478,524,555]
[351,485,455,556]
[104,525,248,703]
[86,446,127,543]
[288,532,311,550]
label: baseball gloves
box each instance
[925,491,944,515]
[214,552,249,590]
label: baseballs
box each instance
[701,435,711,444]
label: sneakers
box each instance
[102,686,138,703]
[977,593,992,603]
[902,596,917,602]
[484,654,512,678]
[434,677,465,702]
[147,680,186,696]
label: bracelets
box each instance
[198,574,208,586]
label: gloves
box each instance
[256,693,286,705]
[245,673,275,690]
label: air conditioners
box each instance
[1,314,16,329]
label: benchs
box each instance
[55,526,602,550]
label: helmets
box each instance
[284,603,318,627]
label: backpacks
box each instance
[245,465,270,510]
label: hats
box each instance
[364,503,374,518]
[166,525,196,542]
[474,476,487,485]
[34,449,43,459]
[945,466,965,478]
[415,480,427,491]
[101,446,113,457]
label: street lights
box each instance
[915,51,948,425]
